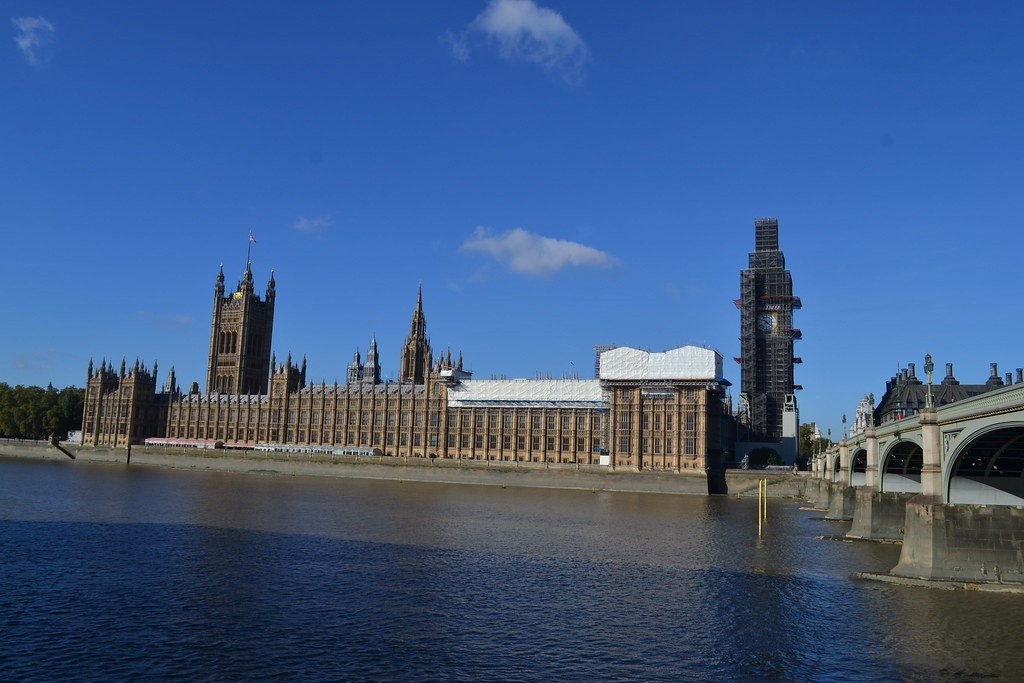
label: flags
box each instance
[250,235,256,242]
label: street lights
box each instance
[827,428,832,448]
[924,352,938,410]
[841,414,847,440]
[868,392,875,428]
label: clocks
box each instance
[756,313,777,331]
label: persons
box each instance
[949,399,955,403]
[792,462,798,475]
